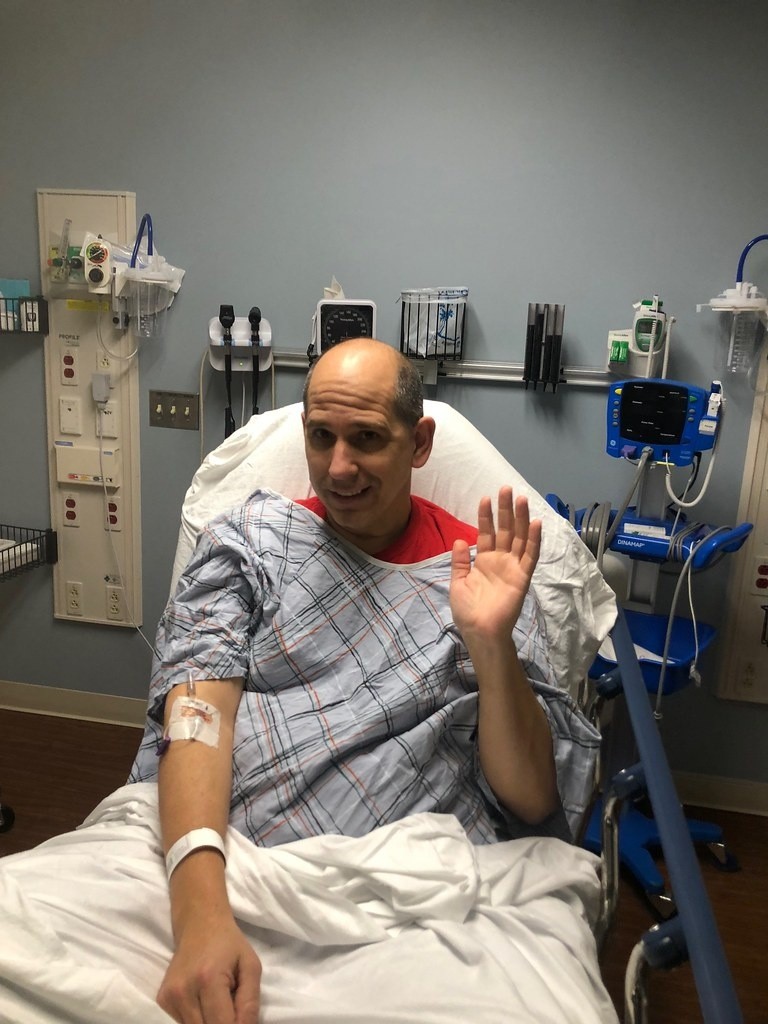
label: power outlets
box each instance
[106,586,124,621]
[735,657,758,695]
[59,346,123,532]
[66,581,83,615]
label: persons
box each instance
[154,338,602,1024]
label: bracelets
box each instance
[166,827,227,881]
